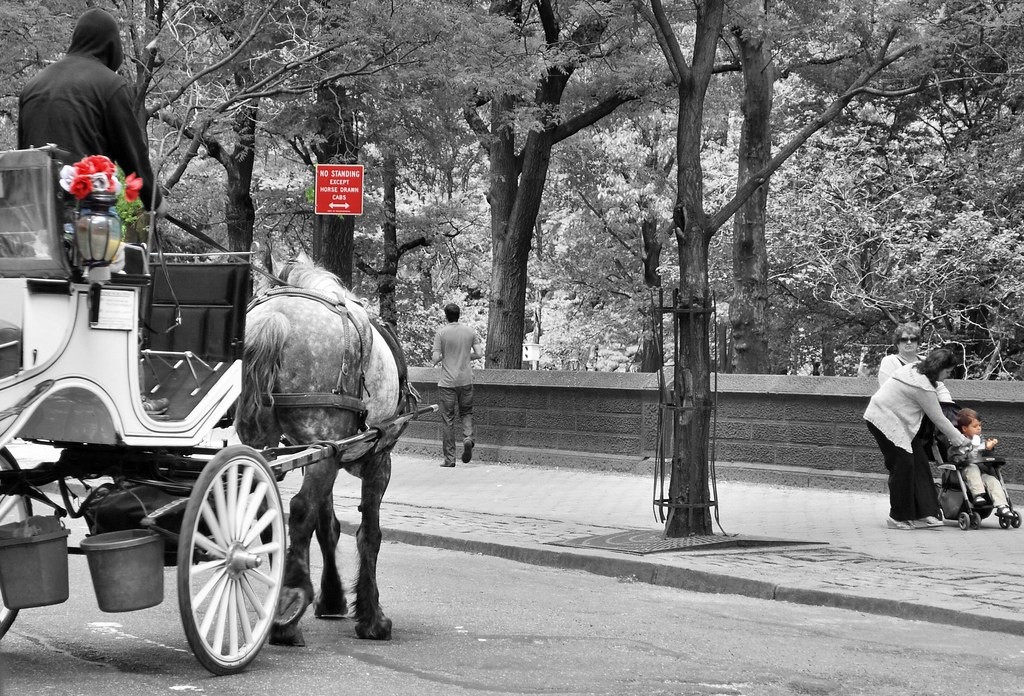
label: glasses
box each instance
[899,337,919,342]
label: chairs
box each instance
[0,143,167,286]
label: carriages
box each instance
[0,142,441,677]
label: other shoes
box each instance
[440,463,455,467]
[461,438,473,464]
[141,396,169,415]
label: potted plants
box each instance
[109,162,145,273]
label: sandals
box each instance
[973,496,987,505]
[994,507,1014,518]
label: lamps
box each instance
[75,194,121,271]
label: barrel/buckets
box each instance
[0,481,71,609]
[81,486,164,613]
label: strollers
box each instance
[917,401,1023,530]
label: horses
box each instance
[232,245,405,648]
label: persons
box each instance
[430,303,483,467]
[862,325,1020,531]
[17,8,170,417]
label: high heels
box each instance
[887,515,916,529]
[914,515,943,528]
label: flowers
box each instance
[59,155,144,203]
